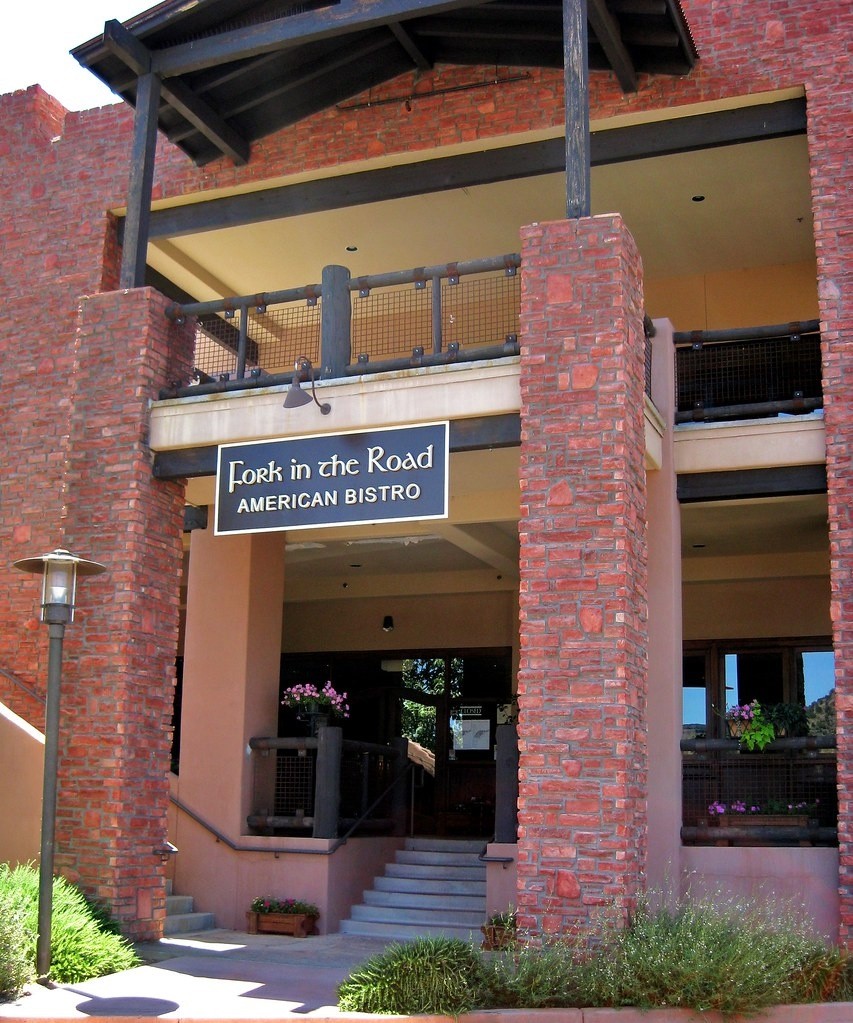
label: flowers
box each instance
[483,908,517,925]
[708,798,822,815]
[281,679,350,720]
[250,895,319,914]
[725,698,809,745]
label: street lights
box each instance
[11,548,107,983]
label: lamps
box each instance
[383,616,394,633]
[283,355,330,417]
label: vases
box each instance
[726,719,762,740]
[716,815,812,847]
[481,922,517,950]
[778,727,786,737]
[305,702,332,718]
[245,912,320,937]
